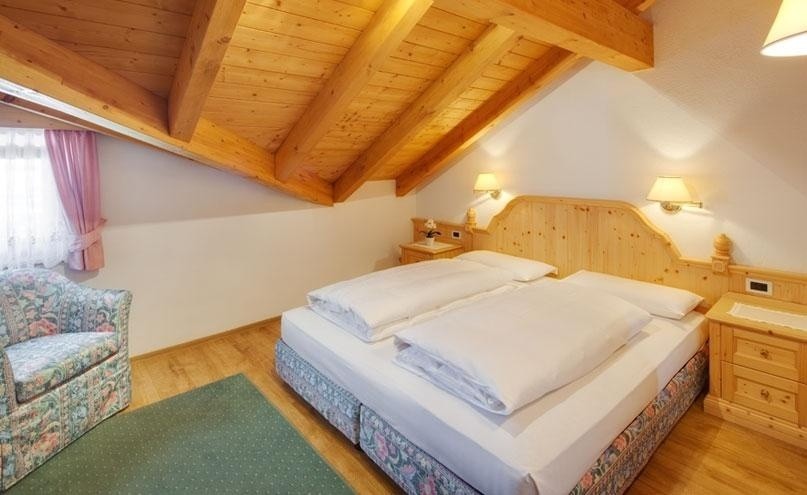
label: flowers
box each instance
[416,217,443,239]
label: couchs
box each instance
[1,266,135,493]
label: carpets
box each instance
[1,370,364,495]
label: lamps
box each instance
[469,168,504,202]
[757,1,807,61]
[645,171,706,216]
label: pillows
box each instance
[560,268,706,319]
[454,244,561,282]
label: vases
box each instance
[424,235,438,248]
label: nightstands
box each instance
[397,234,465,265]
[704,285,807,449]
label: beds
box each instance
[275,192,735,495]
